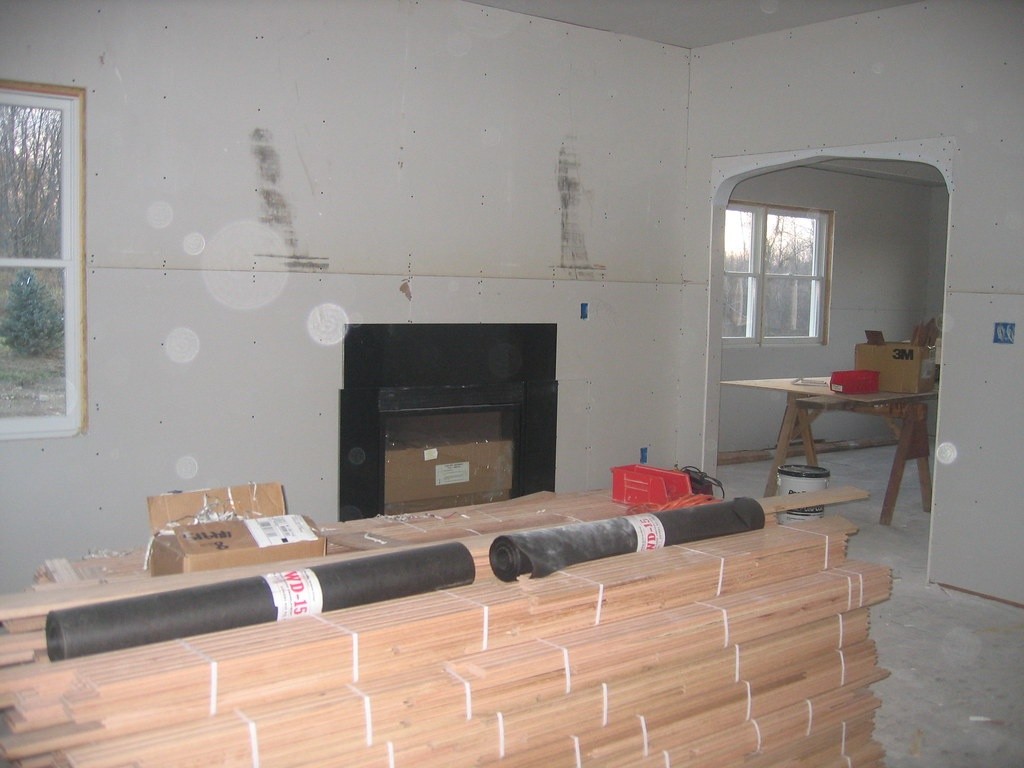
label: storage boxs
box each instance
[147,481,326,578]
[855,342,936,394]
[385,440,513,505]
[830,370,881,395]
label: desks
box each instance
[720,376,940,524]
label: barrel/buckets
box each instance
[776,464,830,524]
